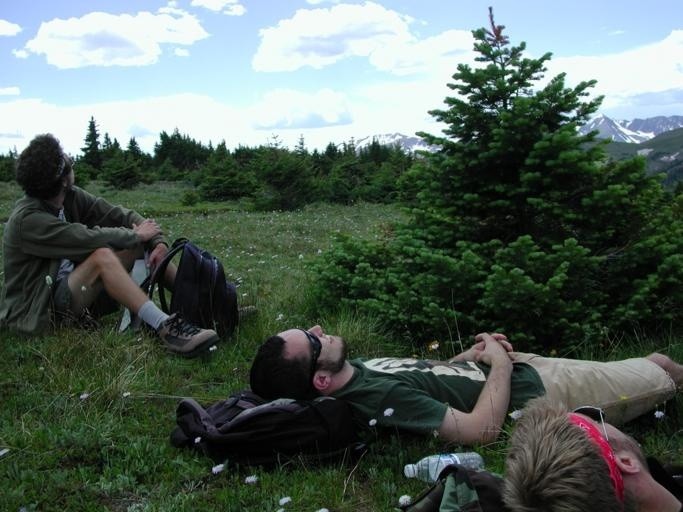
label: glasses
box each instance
[571,403,611,455]
[300,327,322,387]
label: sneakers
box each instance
[154,310,220,358]
[234,302,259,322]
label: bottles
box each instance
[403,451,485,484]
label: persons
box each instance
[500,395,683,512]
[0,135,257,360]
[250,325,683,447]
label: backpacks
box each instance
[172,391,361,469]
[146,238,238,340]
